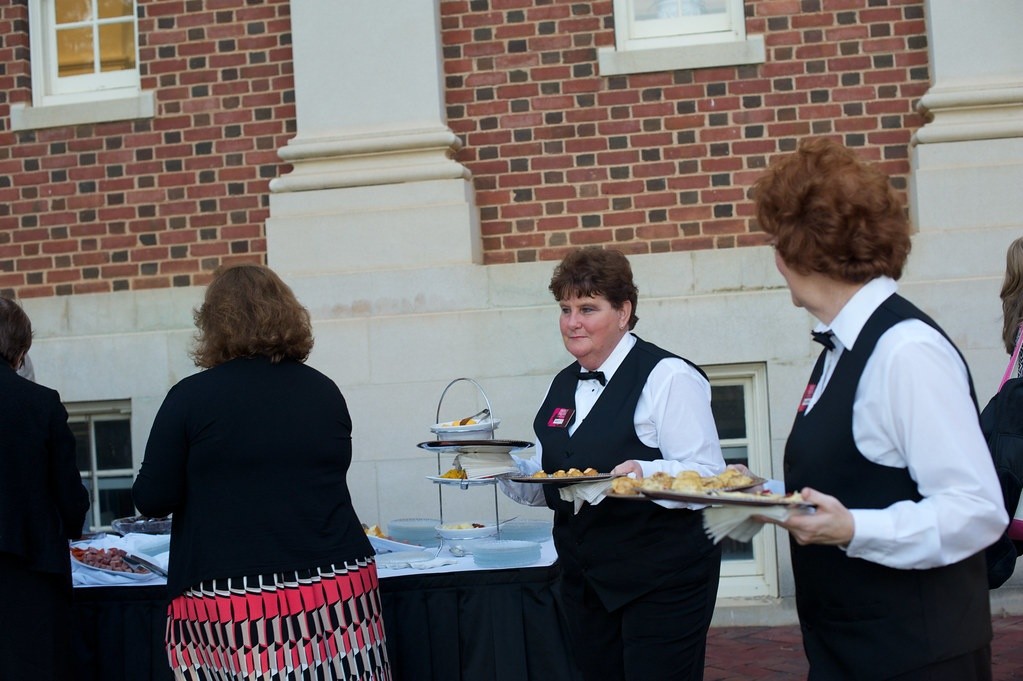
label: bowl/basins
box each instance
[388,517,441,546]
[111,515,173,536]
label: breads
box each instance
[533,467,756,496]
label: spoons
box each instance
[448,547,473,557]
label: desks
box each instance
[70,528,568,681]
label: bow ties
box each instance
[811,330,837,349]
[576,371,605,385]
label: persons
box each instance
[727,139,1011,681]
[493,245,728,681]
[130,264,391,681]
[0,297,88,681]
[999,236,1023,381]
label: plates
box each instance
[416,439,535,454]
[426,476,496,482]
[630,486,816,507]
[429,419,500,432]
[510,473,614,482]
[367,534,424,553]
[435,520,504,538]
[70,546,158,580]
[601,475,768,502]
[474,520,552,568]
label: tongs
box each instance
[116,548,168,578]
[460,408,489,425]
